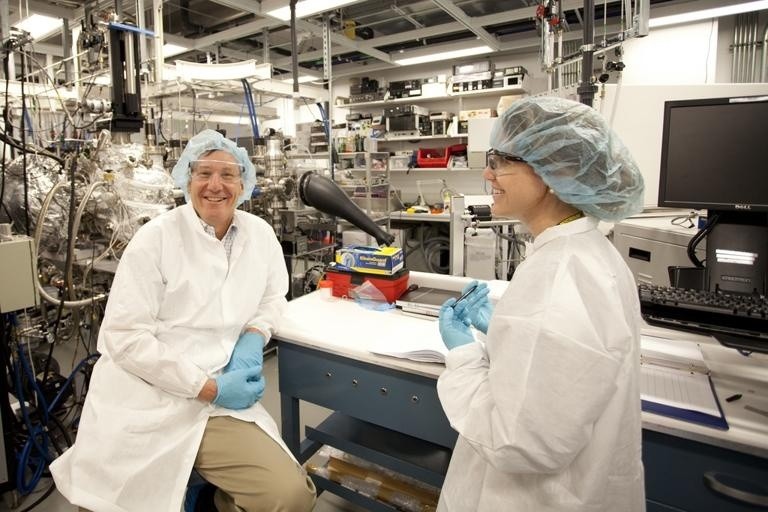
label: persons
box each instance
[435,98,646,512]
[48,128,317,512]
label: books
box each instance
[395,287,462,317]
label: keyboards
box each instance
[636,281,768,354]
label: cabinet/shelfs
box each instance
[334,85,530,280]
[613,223,706,288]
[272,270,768,512]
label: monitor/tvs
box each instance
[657,94,768,210]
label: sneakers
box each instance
[183,483,217,511]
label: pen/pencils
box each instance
[726,394,743,401]
[450,286,477,309]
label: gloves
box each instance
[213,365,265,410]
[224,332,265,382]
[462,281,493,334]
[439,297,475,350]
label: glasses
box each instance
[189,160,245,183]
[486,148,528,176]
